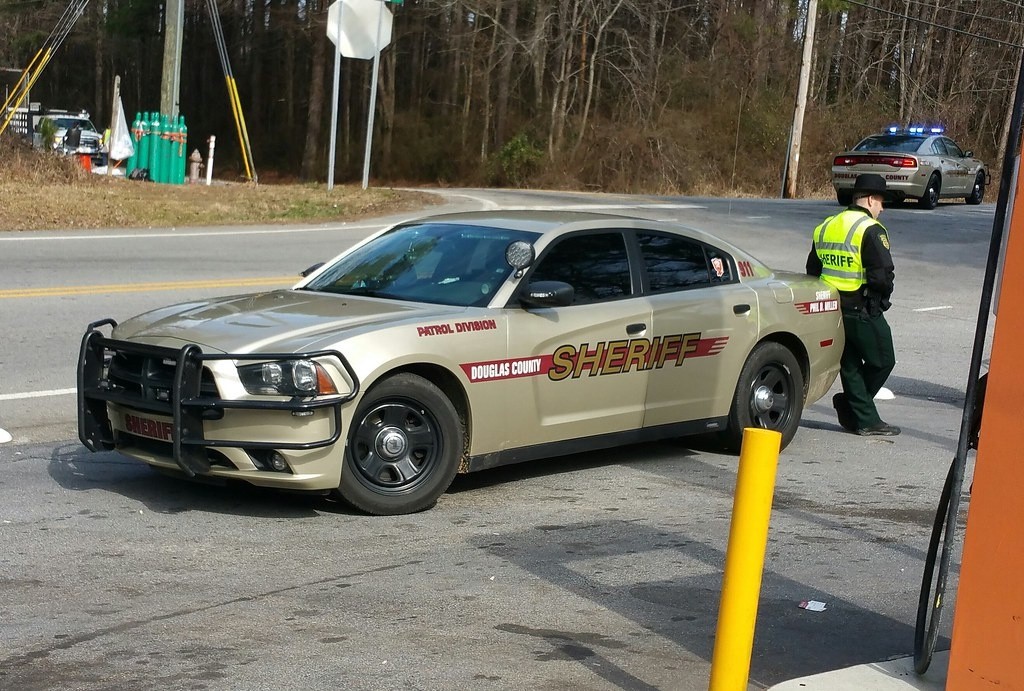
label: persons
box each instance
[806,174,902,437]
[65,122,81,151]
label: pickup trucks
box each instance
[6,106,105,168]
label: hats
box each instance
[851,173,887,194]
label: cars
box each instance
[830,126,992,212]
[71,209,847,519]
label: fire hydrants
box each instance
[188,148,205,184]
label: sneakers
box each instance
[833,392,851,431]
[856,420,901,436]
